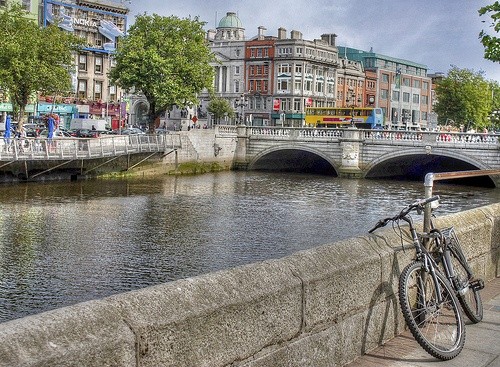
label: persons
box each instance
[162,122,208,132]
[313,110,489,142]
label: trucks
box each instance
[70,118,114,135]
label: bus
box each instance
[304,107,384,129]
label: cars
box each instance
[11,121,74,138]
[66,128,170,137]
[40,130,77,138]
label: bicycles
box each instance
[368,194,485,360]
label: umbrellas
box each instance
[3,115,12,144]
[48,115,55,138]
